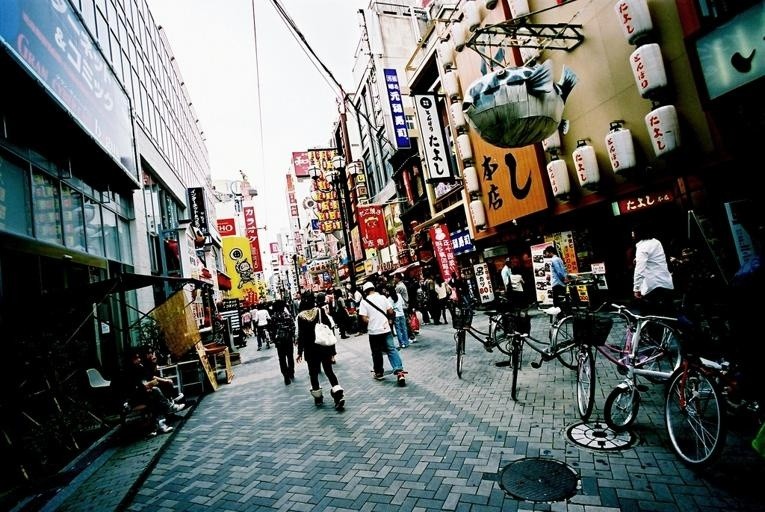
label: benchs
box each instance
[119,383,151,430]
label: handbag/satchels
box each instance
[506,275,513,296]
[444,282,453,297]
[313,307,338,347]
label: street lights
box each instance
[308,156,363,294]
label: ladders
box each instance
[681,210,729,310]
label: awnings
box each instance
[389,262,420,275]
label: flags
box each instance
[354,206,387,249]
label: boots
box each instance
[329,384,346,411]
[309,386,324,406]
[170,392,198,412]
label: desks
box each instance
[157,345,231,396]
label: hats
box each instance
[361,282,376,292]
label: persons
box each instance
[293,290,345,410]
[118,350,186,436]
[501,253,530,318]
[545,245,573,321]
[632,222,677,342]
[241,273,475,384]
[136,342,184,403]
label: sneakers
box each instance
[420,320,449,326]
[338,332,364,338]
[373,372,386,380]
[256,344,270,351]
[396,370,406,387]
[395,338,418,348]
[283,369,295,386]
[165,400,187,415]
[331,359,336,364]
[154,417,174,435]
